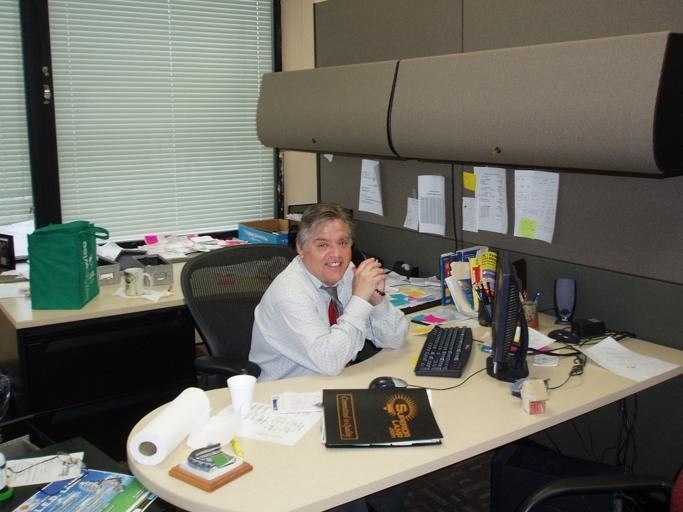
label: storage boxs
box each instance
[237,217,302,247]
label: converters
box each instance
[572,317,606,338]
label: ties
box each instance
[328,298,340,327]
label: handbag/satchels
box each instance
[26,221,110,310]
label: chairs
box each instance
[180,242,298,390]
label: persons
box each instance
[246,199,410,384]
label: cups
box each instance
[226,374,258,417]
[124,266,153,297]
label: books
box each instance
[437,248,500,318]
[136,228,249,262]
[318,385,443,450]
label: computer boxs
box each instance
[490,444,624,512]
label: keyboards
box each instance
[414,326,472,378]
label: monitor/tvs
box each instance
[486,250,530,382]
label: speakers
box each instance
[554,278,576,325]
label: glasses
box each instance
[8,451,89,496]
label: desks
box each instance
[127,295,683,512]
[1,240,451,442]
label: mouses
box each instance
[368,376,408,391]
[548,330,580,343]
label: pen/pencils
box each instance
[475,282,492,327]
[518,290,540,303]
[360,252,367,259]
[231,438,243,457]
